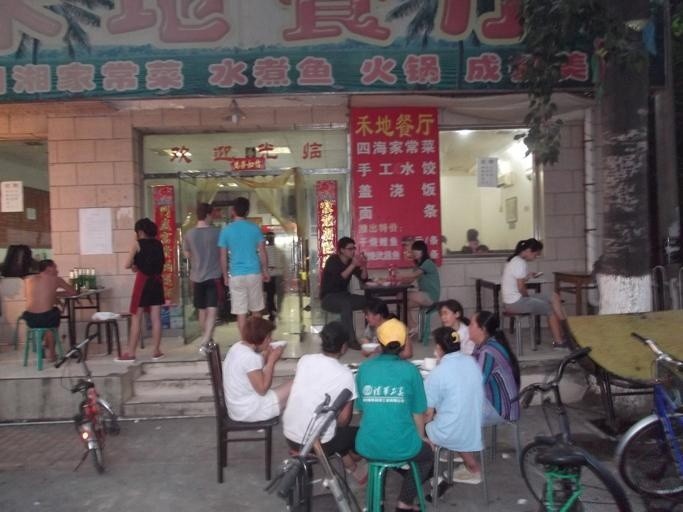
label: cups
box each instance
[423,357,436,369]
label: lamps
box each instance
[220,96,248,127]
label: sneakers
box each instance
[40,338,58,365]
[353,463,369,489]
[552,339,571,351]
[199,340,217,357]
[442,463,483,486]
[437,448,464,465]
[151,351,165,361]
[395,503,428,512]
[113,352,137,364]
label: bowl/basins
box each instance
[361,342,379,354]
[267,339,288,351]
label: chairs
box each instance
[199,341,276,484]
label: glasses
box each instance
[342,245,357,251]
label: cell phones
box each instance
[534,272,543,278]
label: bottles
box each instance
[69,267,96,294]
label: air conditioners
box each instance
[496,171,514,191]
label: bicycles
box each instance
[55,333,122,475]
[610,330,683,499]
[507,345,633,512]
[263,388,363,512]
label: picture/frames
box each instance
[504,194,518,224]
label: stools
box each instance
[481,421,525,479]
[415,306,440,343]
[10,313,36,352]
[19,327,63,370]
[427,441,490,511]
[78,317,119,360]
[113,310,147,350]
[365,455,426,512]
[288,448,348,512]
[500,309,537,357]
[320,309,341,327]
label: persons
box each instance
[22,259,79,363]
[263,232,288,321]
[388,241,440,338]
[216,197,271,336]
[320,237,371,350]
[113,218,165,363]
[440,229,488,256]
[500,238,568,351]
[183,203,221,356]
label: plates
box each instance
[365,278,395,287]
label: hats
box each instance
[374,318,408,349]
